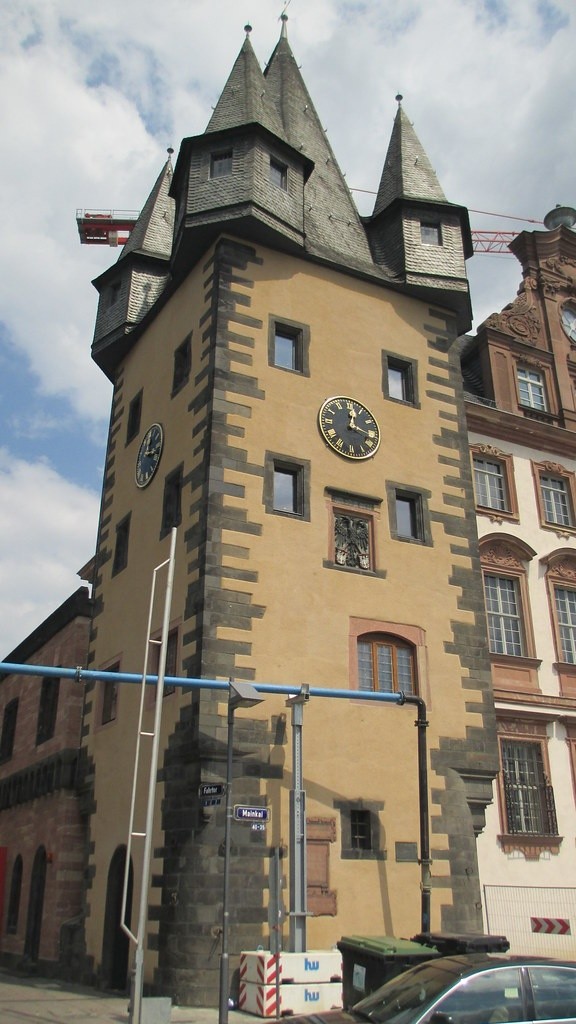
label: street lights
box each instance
[219,679,266,1024]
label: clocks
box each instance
[135,423,162,487]
[319,395,380,458]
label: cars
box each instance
[273,956,576,1024]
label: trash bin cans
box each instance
[336,934,442,1009]
[411,932,511,956]
